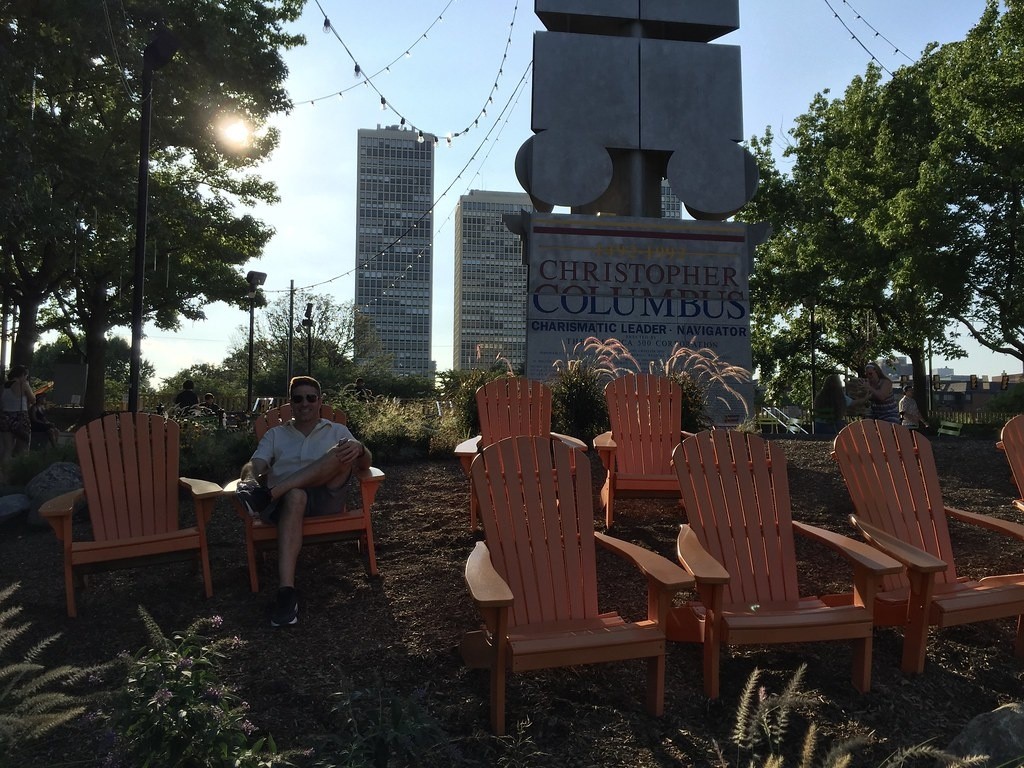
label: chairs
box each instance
[39,412,224,618]
[224,403,386,593]
[593,373,715,530]
[831,419,1024,674]
[785,418,804,434]
[454,376,588,531]
[666,430,903,695]
[937,419,963,436]
[997,414,1024,514]
[462,435,695,738]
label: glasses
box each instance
[291,394,320,403]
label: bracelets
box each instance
[357,445,366,458]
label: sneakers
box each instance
[271,586,297,626]
[236,485,271,517]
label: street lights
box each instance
[242,270,270,417]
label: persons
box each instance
[199,393,220,416]
[898,385,930,433]
[814,362,900,434]
[237,377,372,627]
[355,377,372,402]
[173,379,199,409]
[0,365,60,461]
